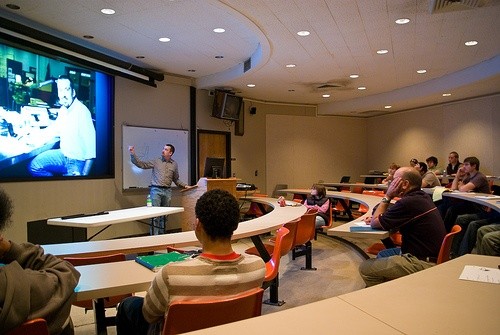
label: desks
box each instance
[177,296,406,335]
[73,259,160,335]
[276,188,397,250]
[46,206,184,243]
[337,253,500,335]
[359,175,496,194]
[316,182,500,222]
[40,194,318,306]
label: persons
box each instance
[410,159,418,167]
[0,189,80,335]
[446,151,463,176]
[426,157,444,174]
[450,212,500,258]
[117,190,266,335]
[27,75,96,178]
[303,185,330,227]
[358,167,446,288]
[415,162,441,187]
[382,164,399,183]
[476,224,500,257]
[444,157,489,233]
[128,144,194,236]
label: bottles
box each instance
[146,194,152,207]
[279,197,285,207]
[442,170,447,185]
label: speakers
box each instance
[212,90,243,121]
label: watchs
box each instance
[381,197,390,204]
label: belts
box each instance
[152,185,170,189]
[417,256,436,263]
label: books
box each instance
[475,195,500,199]
[349,222,378,232]
[134,251,191,272]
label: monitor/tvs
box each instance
[204,157,225,179]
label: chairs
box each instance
[0,174,496,335]
